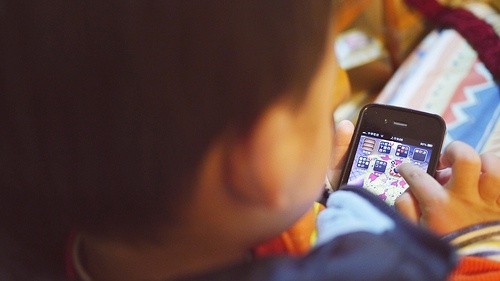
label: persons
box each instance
[0,0,500,280]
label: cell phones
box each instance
[336,103,446,209]
